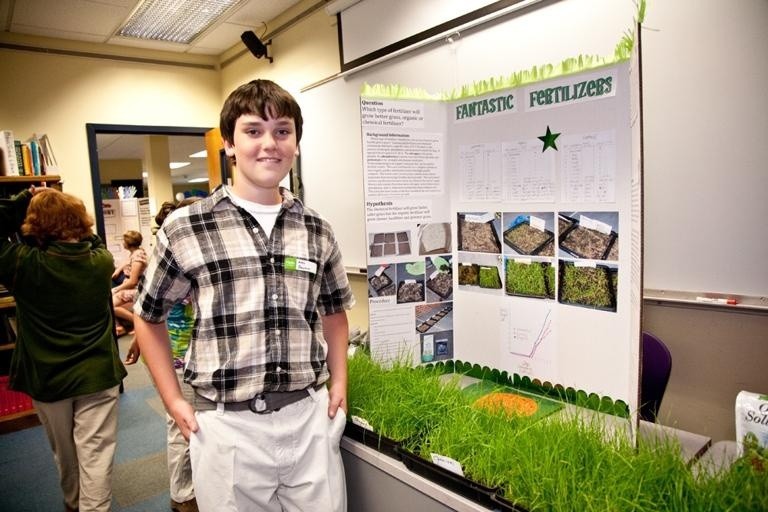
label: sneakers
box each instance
[171,497,198,512]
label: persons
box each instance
[112,228,148,336]
[0,184,129,511]
[122,196,204,511]
[133,77,357,512]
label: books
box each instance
[0,130,62,176]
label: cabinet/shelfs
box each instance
[0,174,63,424]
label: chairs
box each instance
[641,329,672,423]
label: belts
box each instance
[191,382,326,414]
